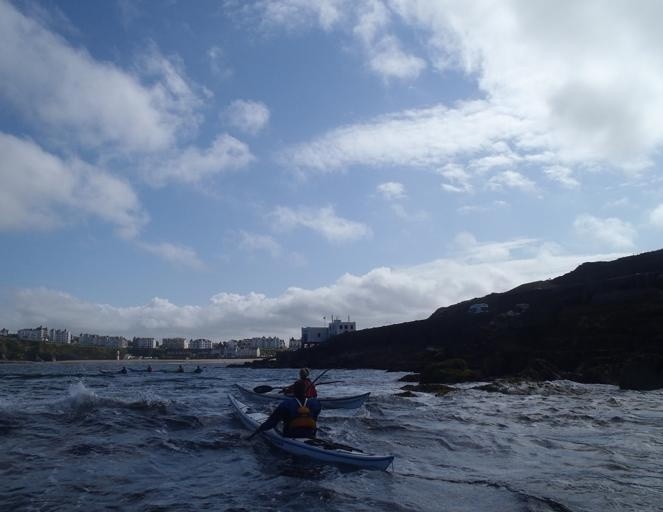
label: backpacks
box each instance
[285,398,316,438]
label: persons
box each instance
[176,365,184,373]
[284,368,318,398]
[147,365,153,373]
[193,365,202,374]
[118,367,127,374]
[256,380,321,441]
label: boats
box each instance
[226,392,394,473]
[97,367,200,376]
[236,381,373,411]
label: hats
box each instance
[300,368,310,377]
